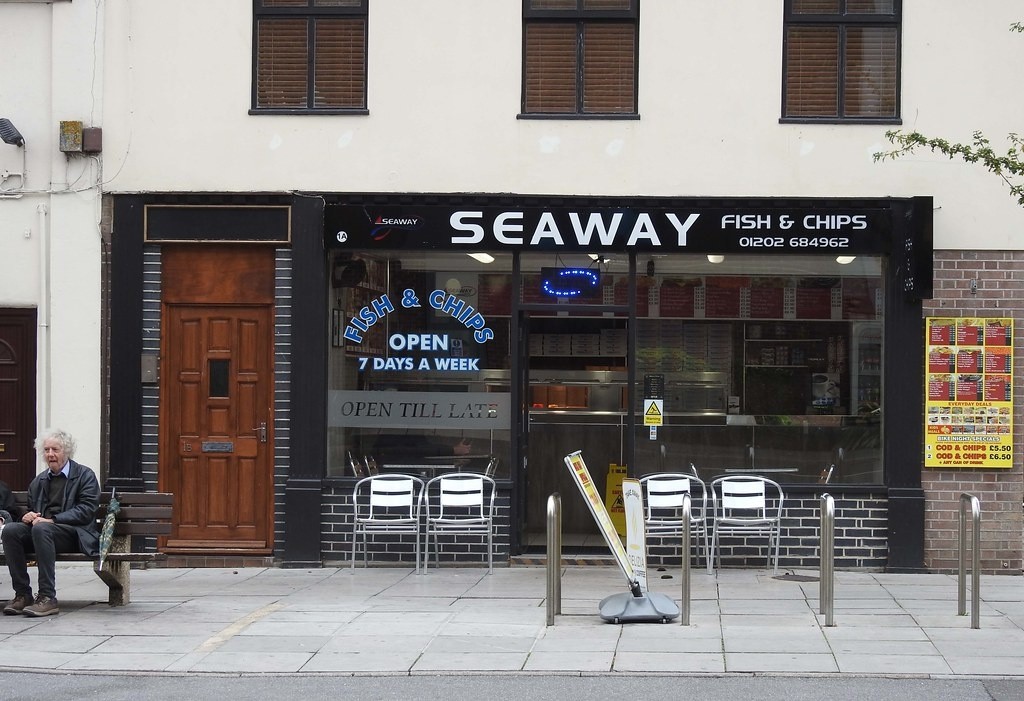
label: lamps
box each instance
[0,118,23,148]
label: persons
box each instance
[1,430,100,618]
[371,428,471,477]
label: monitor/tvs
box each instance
[742,365,813,416]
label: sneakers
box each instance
[23,593,59,615]
[3,593,34,614]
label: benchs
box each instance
[0,491,175,608]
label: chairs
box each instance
[708,475,784,576]
[638,471,713,571]
[422,473,497,575]
[347,474,423,575]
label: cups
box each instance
[812,374,835,398]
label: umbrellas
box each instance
[98,487,121,572]
